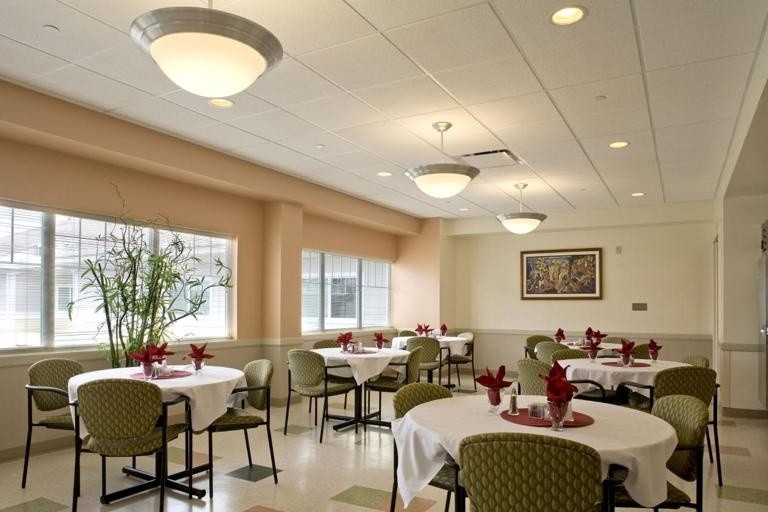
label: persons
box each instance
[528,273,543,293]
[544,271,553,289]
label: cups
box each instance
[623,354,630,368]
[376,341,384,351]
[192,357,204,375]
[629,354,632,366]
[152,363,157,379]
[546,401,568,432]
[341,343,347,351]
[587,348,598,361]
[441,330,446,337]
[565,401,574,421]
[617,353,623,366]
[142,365,152,380]
[649,350,657,361]
[487,389,501,412]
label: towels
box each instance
[538,360,579,423]
[646,339,663,361]
[148,343,176,365]
[182,342,217,370]
[422,324,434,336]
[585,327,607,346]
[554,327,566,344]
[611,338,641,365]
[440,323,448,336]
[371,332,390,350]
[127,349,166,377]
[474,365,513,405]
[580,339,604,360]
[415,324,423,335]
[336,332,354,352]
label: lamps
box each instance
[404,122,480,198]
[130,1,282,98]
[496,184,548,235]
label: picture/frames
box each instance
[520,248,603,300]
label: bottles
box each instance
[507,388,520,416]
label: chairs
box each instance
[284,349,359,444]
[407,337,443,386]
[517,359,607,408]
[400,331,418,337]
[439,332,477,389]
[682,355,709,368]
[604,396,709,512]
[534,342,570,366]
[457,433,603,512]
[364,346,425,432]
[22,358,106,497]
[406,337,451,391]
[309,340,357,426]
[525,335,555,358]
[390,383,465,512]
[551,349,591,361]
[67,378,192,512]
[617,367,724,487]
[193,358,278,476]
[603,338,630,344]
